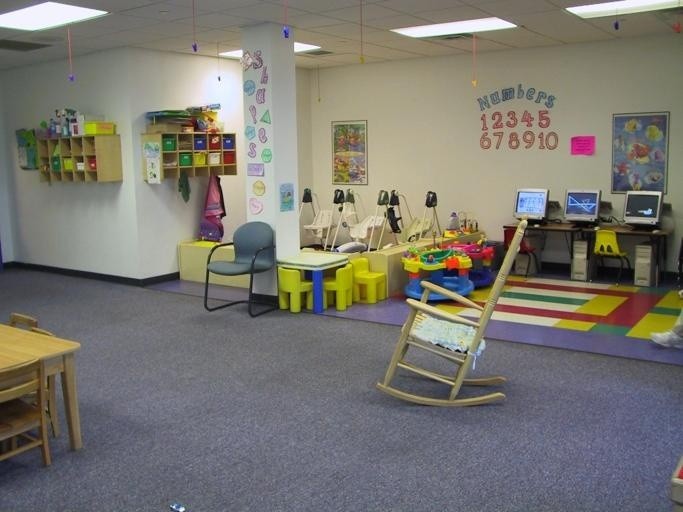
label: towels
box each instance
[204,172,227,224]
[176,167,192,205]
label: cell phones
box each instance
[170,503,186,512]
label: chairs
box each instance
[501,226,541,280]
[202,221,276,318]
[323,260,354,312]
[7,325,61,452]
[589,227,634,290]
[373,213,530,410]
[277,266,314,313]
[0,357,52,469]
[10,312,37,334]
[351,256,389,305]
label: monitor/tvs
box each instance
[513,189,550,224]
[623,191,663,231]
[563,190,601,227]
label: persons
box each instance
[648,308,683,350]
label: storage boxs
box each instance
[159,130,236,166]
[50,158,96,171]
[83,120,114,136]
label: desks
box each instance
[502,219,674,290]
[275,252,349,314]
[0,323,86,452]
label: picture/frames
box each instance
[330,120,369,186]
[609,111,670,197]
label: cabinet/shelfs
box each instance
[36,133,124,184]
[139,130,237,187]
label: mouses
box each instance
[594,226,600,231]
[652,230,660,234]
[534,224,540,228]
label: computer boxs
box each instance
[570,239,597,282]
[634,241,661,287]
[515,234,541,276]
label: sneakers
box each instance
[649,330,683,349]
